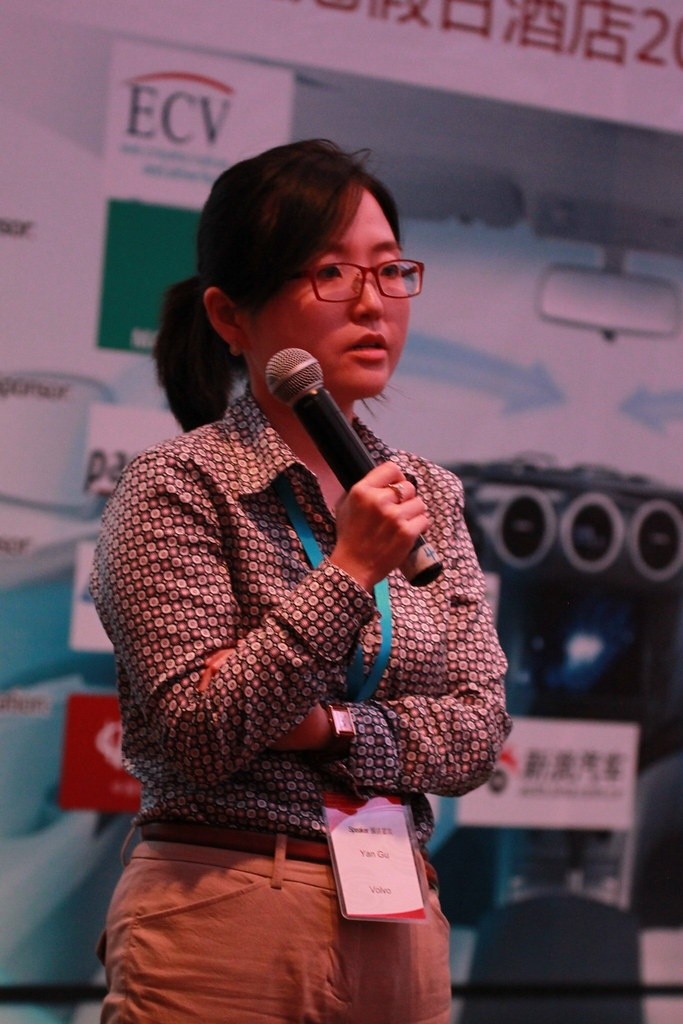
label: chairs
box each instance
[459,893,643,1024]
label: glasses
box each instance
[279,259,424,302]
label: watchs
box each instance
[320,699,356,756]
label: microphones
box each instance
[266,348,443,587]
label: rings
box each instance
[384,483,403,504]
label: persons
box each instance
[88,139,514,1023]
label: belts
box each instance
[141,820,440,882]
